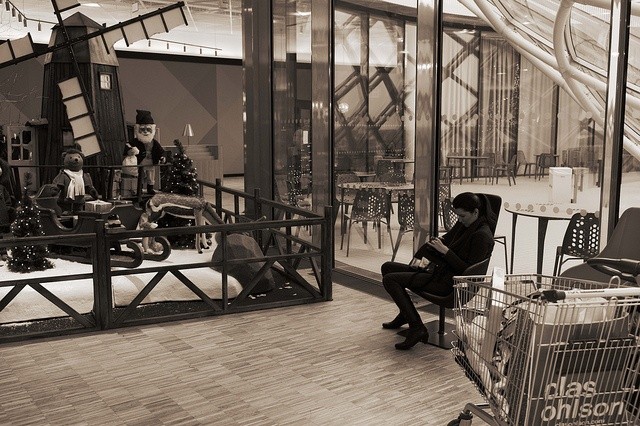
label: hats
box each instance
[136,109,154,124]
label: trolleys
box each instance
[447,273,640,426]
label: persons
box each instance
[123,108,167,197]
[381,191,496,351]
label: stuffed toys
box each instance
[51,148,100,218]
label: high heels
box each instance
[396,329,430,349]
[383,312,408,328]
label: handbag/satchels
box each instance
[507,298,629,426]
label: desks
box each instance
[503,199,601,291]
[336,181,414,244]
[446,156,488,185]
[536,154,560,178]
[380,157,414,183]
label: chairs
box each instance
[441,199,508,280]
[335,172,361,223]
[516,150,537,177]
[390,193,446,262]
[533,152,556,182]
[551,212,600,289]
[438,183,451,224]
[341,186,386,256]
[372,155,383,182]
[399,193,502,350]
[491,154,517,186]
[371,159,409,215]
[439,168,449,182]
